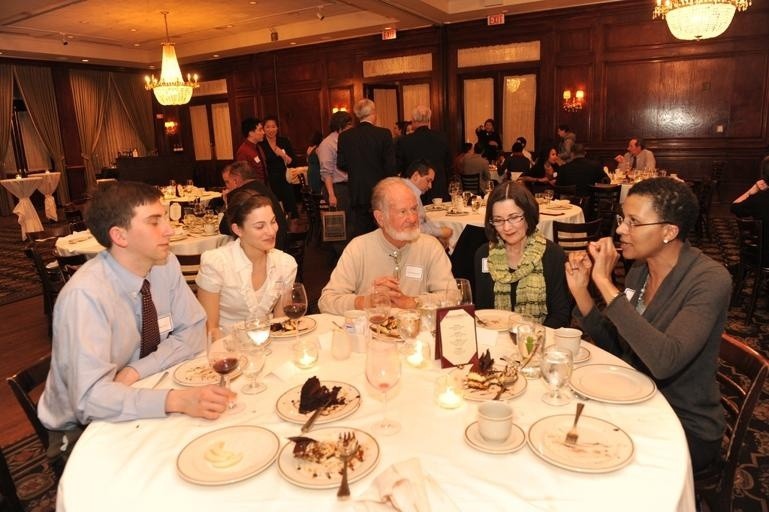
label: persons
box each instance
[36,180,236,482]
[195,189,299,343]
[221,99,657,252]
[564,177,733,475]
[723,152,769,281]
[317,177,463,315]
[468,181,576,329]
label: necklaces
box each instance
[637,275,649,313]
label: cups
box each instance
[444,279,471,308]
[204,209,215,235]
[553,327,582,355]
[476,402,514,443]
[398,309,423,347]
[432,197,443,206]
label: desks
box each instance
[60,307,694,512]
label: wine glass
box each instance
[507,313,545,381]
[535,190,554,214]
[540,345,573,406]
[207,314,272,416]
[364,285,401,436]
[284,282,308,350]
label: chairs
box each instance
[7,354,56,443]
[0,152,768,322]
[696,330,768,510]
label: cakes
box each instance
[299,375,332,413]
[466,350,496,390]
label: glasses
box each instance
[616,214,674,229]
[489,214,524,225]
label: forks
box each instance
[335,432,358,499]
[563,403,584,450]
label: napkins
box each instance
[365,464,429,512]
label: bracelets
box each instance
[606,291,621,306]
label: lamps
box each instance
[560,90,584,113]
[144,11,200,106]
[652,0,753,41]
[507,77,520,92]
[165,121,177,136]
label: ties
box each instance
[141,279,161,358]
[632,156,636,168]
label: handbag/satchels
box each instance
[321,211,348,243]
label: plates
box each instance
[170,234,189,242]
[528,413,636,475]
[543,344,592,364]
[270,316,318,339]
[425,205,470,217]
[571,364,657,404]
[176,382,380,488]
[174,352,248,387]
[474,309,522,331]
[447,363,528,453]
